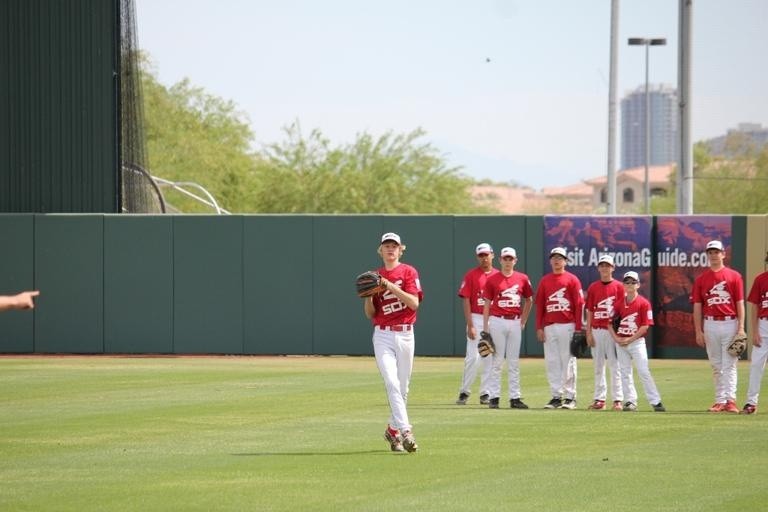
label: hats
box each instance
[381,233,401,246]
[500,246,517,259]
[476,242,494,255]
[706,240,725,251]
[549,247,567,259]
[598,254,614,266]
[623,271,640,282]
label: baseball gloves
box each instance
[355,270,390,300]
[476,330,497,357]
[725,333,751,356]
[569,331,592,360]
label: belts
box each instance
[706,315,735,321]
[494,314,520,319]
[380,324,411,331]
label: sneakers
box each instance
[707,402,757,414]
[383,429,418,452]
[480,394,499,408]
[509,398,528,408]
[588,399,606,410]
[651,402,665,411]
[456,393,469,405]
[544,398,577,409]
[612,401,638,411]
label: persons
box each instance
[476,246,534,409]
[454,240,499,406]
[533,247,584,410]
[583,254,627,412]
[688,240,746,414]
[741,251,768,414]
[606,271,665,412]
[354,232,423,455]
[0,290,41,314]
